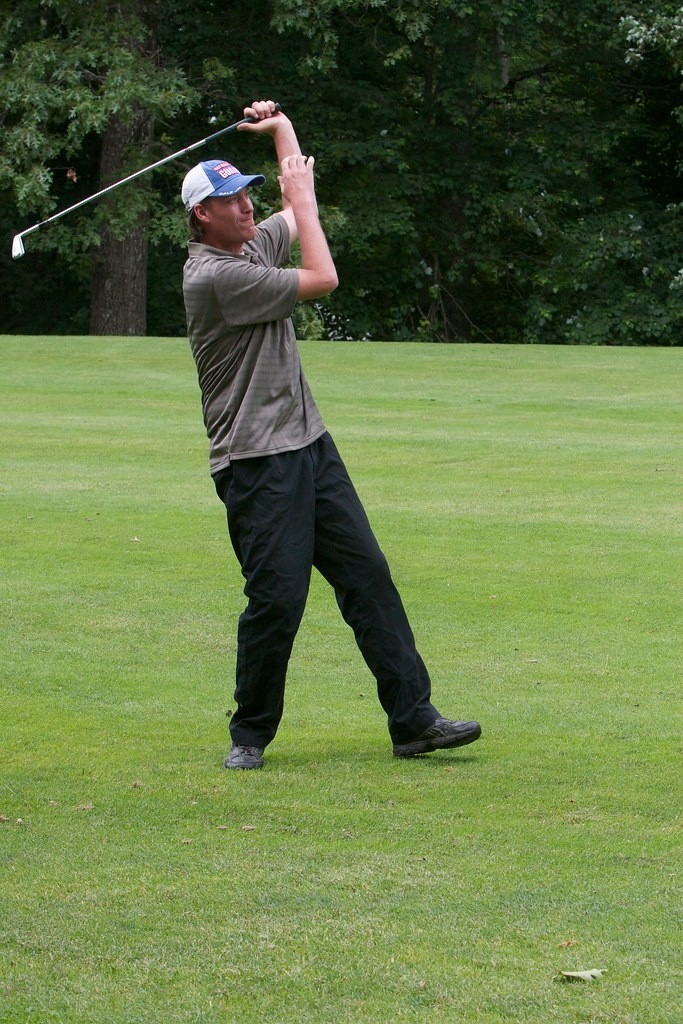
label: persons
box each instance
[181,101,483,771]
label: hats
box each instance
[181,160,266,212]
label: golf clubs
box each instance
[11,102,282,261]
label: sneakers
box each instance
[224,746,264,769]
[393,717,482,755]
